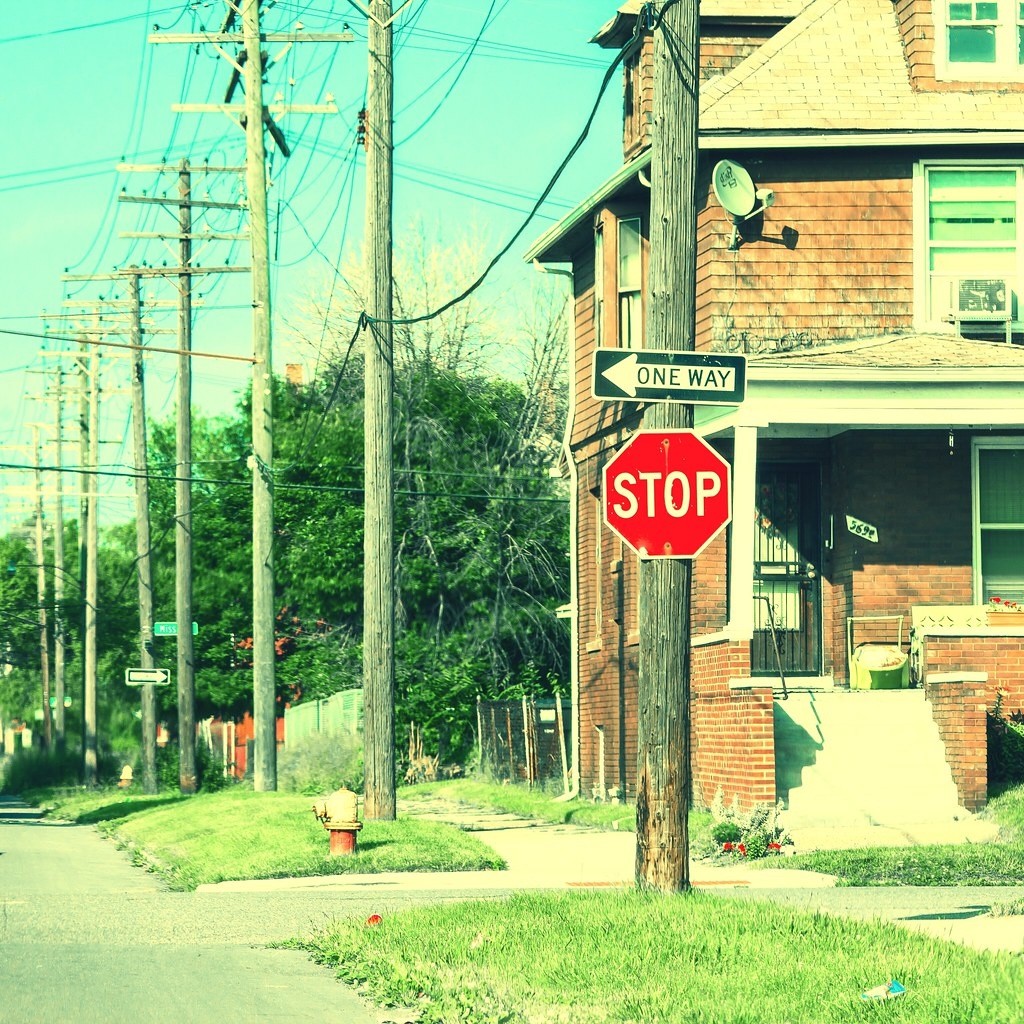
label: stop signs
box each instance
[602,428,731,562]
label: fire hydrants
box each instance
[313,785,363,856]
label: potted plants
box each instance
[984,597,1024,628]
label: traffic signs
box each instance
[593,348,744,406]
[126,668,171,686]
